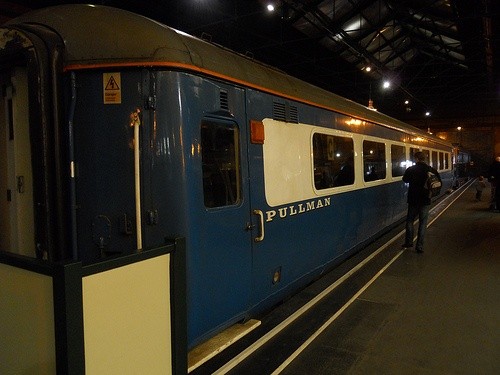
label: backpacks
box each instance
[424,166,442,198]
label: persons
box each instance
[487,157,500,212]
[475,176,486,201]
[401,152,442,256]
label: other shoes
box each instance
[417,249,423,253]
[402,244,413,247]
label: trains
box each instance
[0,0,481,354]
